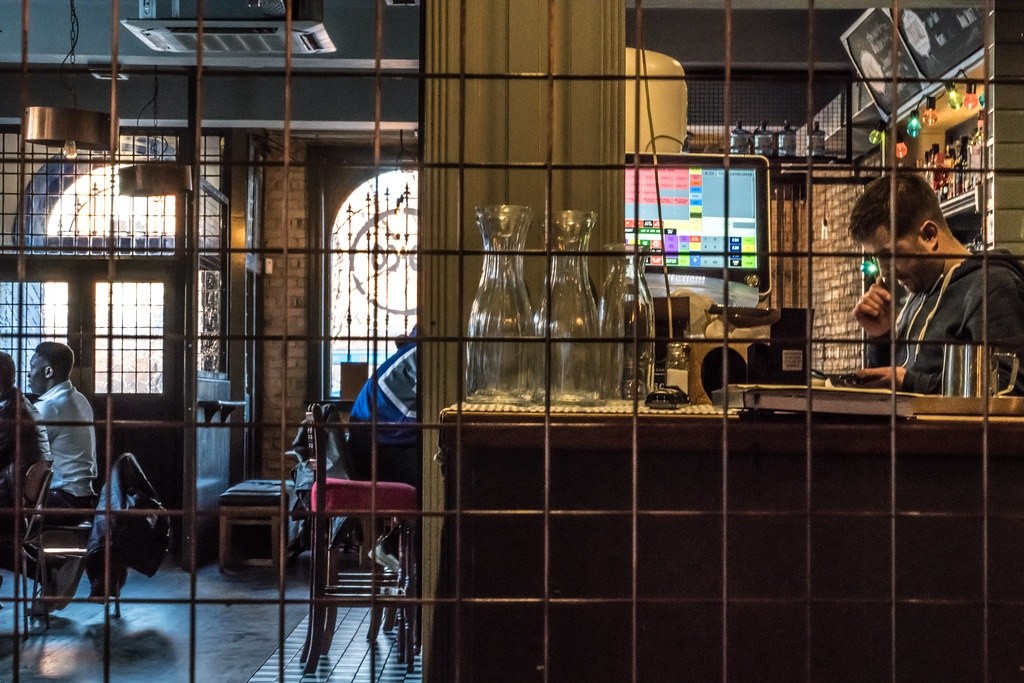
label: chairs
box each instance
[35,453,136,629]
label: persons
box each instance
[28,342,99,533]
[848,173,1024,396]
[345,324,418,572]
[0,352,84,615]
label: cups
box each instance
[942,344,1019,398]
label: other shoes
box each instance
[368,544,399,572]
[53,555,86,610]
[26,583,55,616]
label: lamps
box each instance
[120,66,191,197]
[23,0,115,162]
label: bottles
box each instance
[466,205,535,404]
[533,210,601,406]
[597,244,654,406]
[665,344,692,397]
[729,121,749,153]
[917,126,984,203]
[777,120,796,156]
[811,121,826,156]
[755,121,773,158]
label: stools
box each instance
[220,399,423,674]
